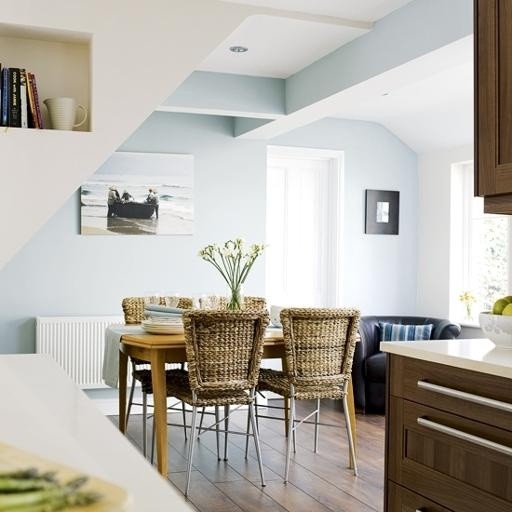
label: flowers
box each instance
[197,236,269,309]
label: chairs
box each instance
[244,308,361,484]
[197,295,268,460]
[122,296,194,443]
[152,310,270,498]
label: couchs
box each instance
[353,315,462,415]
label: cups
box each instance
[43,96,88,131]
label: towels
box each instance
[102,324,145,389]
[144,304,185,317]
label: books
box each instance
[0,63,44,129]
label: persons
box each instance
[105,185,160,220]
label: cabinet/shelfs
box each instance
[380,344,512,512]
[473,0,511,219]
[0,23,92,133]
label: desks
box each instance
[117,326,358,484]
[0,351,196,512]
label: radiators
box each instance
[34,317,187,389]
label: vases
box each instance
[227,283,247,309]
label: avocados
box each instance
[493,299,507,315]
[502,302,512,316]
[505,295,512,302]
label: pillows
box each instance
[376,320,433,353]
